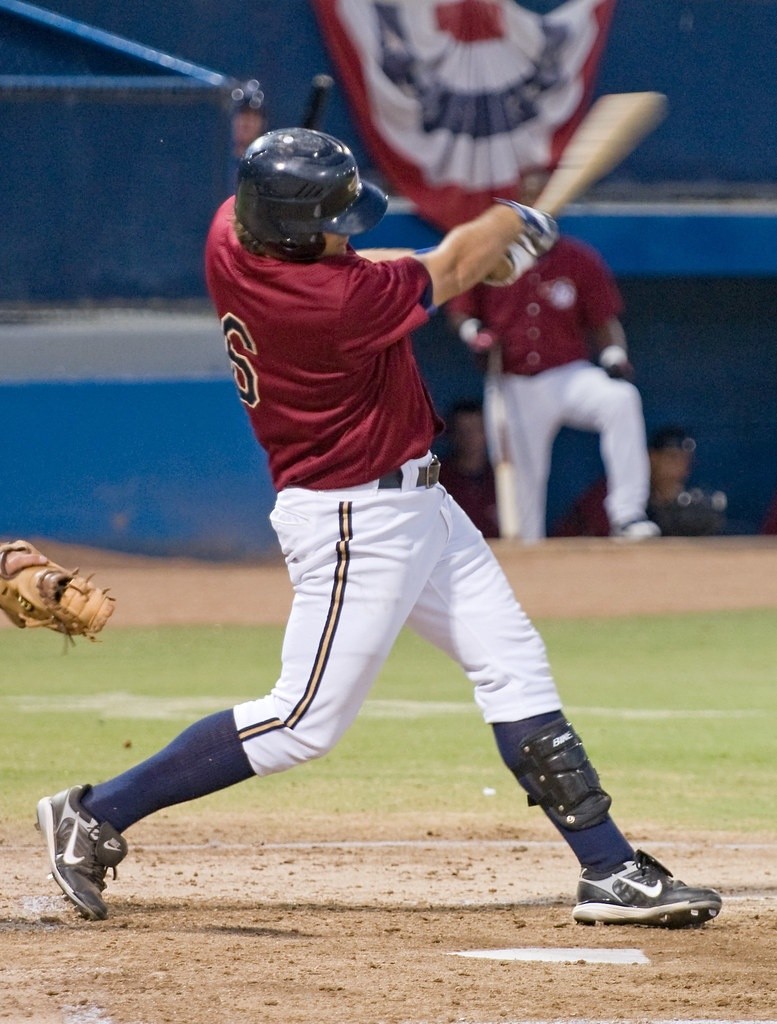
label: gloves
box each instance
[597,345,638,381]
[476,197,559,286]
[457,318,500,354]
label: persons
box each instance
[223,81,721,541]
[32,128,722,927]
[0,539,115,643]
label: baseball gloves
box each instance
[0,536,116,642]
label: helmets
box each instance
[234,126,389,263]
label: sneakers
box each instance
[609,518,662,543]
[572,849,723,926]
[34,783,129,922]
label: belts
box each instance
[379,453,442,489]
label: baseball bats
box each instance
[485,88,672,287]
